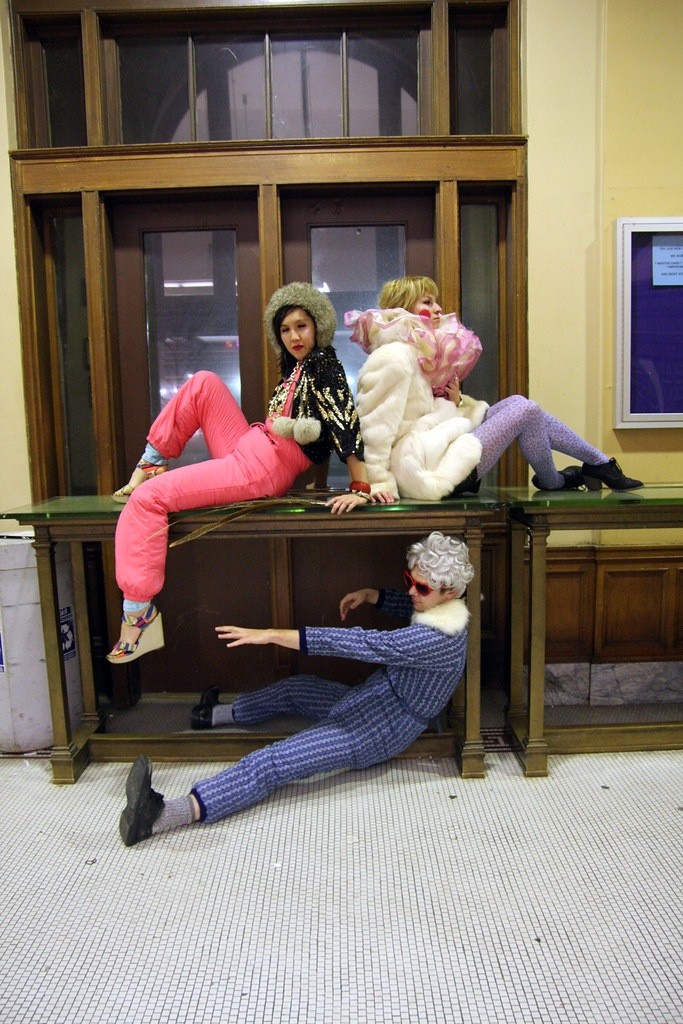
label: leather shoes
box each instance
[190,685,223,729]
[119,755,166,846]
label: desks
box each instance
[0,481,683,785]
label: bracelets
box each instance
[350,489,372,502]
[457,393,464,408]
[348,480,371,494]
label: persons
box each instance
[344,277,645,504]
[119,530,475,847]
[105,280,372,664]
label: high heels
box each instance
[112,458,168,503]
[106,604,165,664]
[532,466,584,490]
[581,457,644,492]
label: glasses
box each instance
[403,570,432,596]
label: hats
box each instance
[263,282,336,359]
